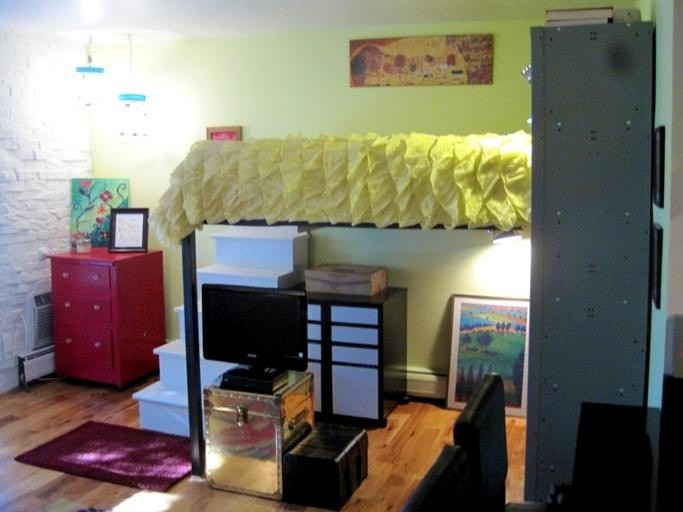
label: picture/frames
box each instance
[654,126,665,207]
[652,224,663,309]
[108,209,149,253]
[207,125,244,140]
[445,295,530,418]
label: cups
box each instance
[75,238,92,254]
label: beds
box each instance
[147,130,532,476]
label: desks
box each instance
[573,376,683,512]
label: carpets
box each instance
[14,421,205,492]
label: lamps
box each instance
[76,38,104,105]
[119,33,146,136]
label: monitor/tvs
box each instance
[453,372,507,512]
[202,284,307,394]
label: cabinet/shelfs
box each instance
[292,282,407,427]
[525,22,656,502]
[46,247,165,392]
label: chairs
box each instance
[454,374,548,512]
